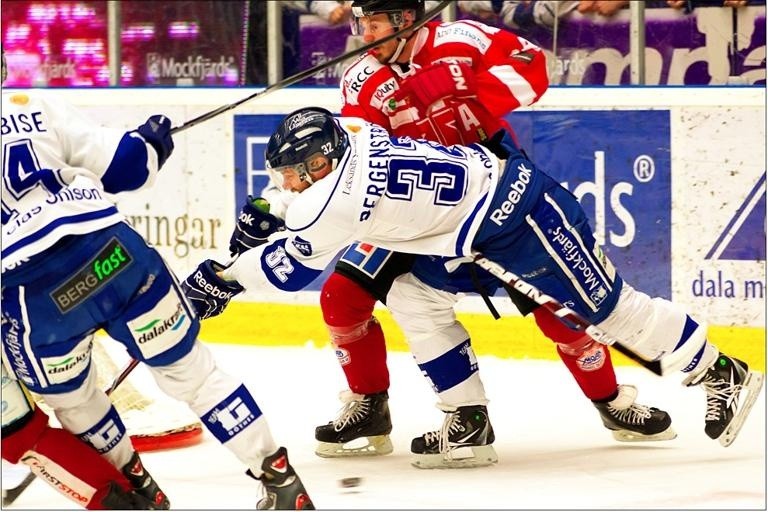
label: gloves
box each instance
[416,94,493,147]
[140,113,175,169]
[179,259,243,321]
[229,194,281,257]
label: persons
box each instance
[1,50,312,510]
[181,106,743,438]
[314,0,670,442]
[247,0,766,87]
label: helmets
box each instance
[265,108,345,188]
[348,0,426,44]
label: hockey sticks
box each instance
[472,253,707,377]
[168,0,456,132]
[3,359,141,508]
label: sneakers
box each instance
[256,446,316,509]
[315,391,393,442]
[103,478,157,509]
[410,402,494,454]
[682,352,748,440]
[593,382,671,435]
[120,450,169,510]
